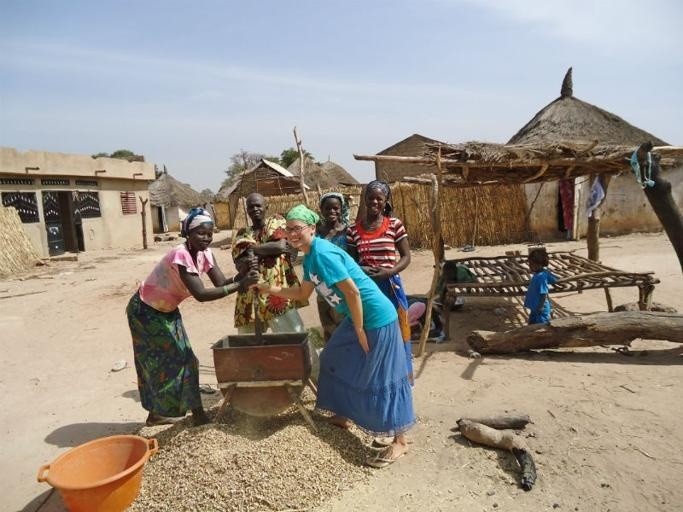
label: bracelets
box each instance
[222,285,228,296]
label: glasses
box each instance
[285,223,312,233]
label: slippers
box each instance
[375,435,413,447]
[365,450,407,468]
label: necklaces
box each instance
[366,221,379,229]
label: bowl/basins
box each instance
[37,435,157,512]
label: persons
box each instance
[345,180,414,388]
[314,192,350,344]
[252,205,415,468]
[524,248,556,324]
[231,194,319,380]
[435,262,476,301]
[127,207,258,427]
